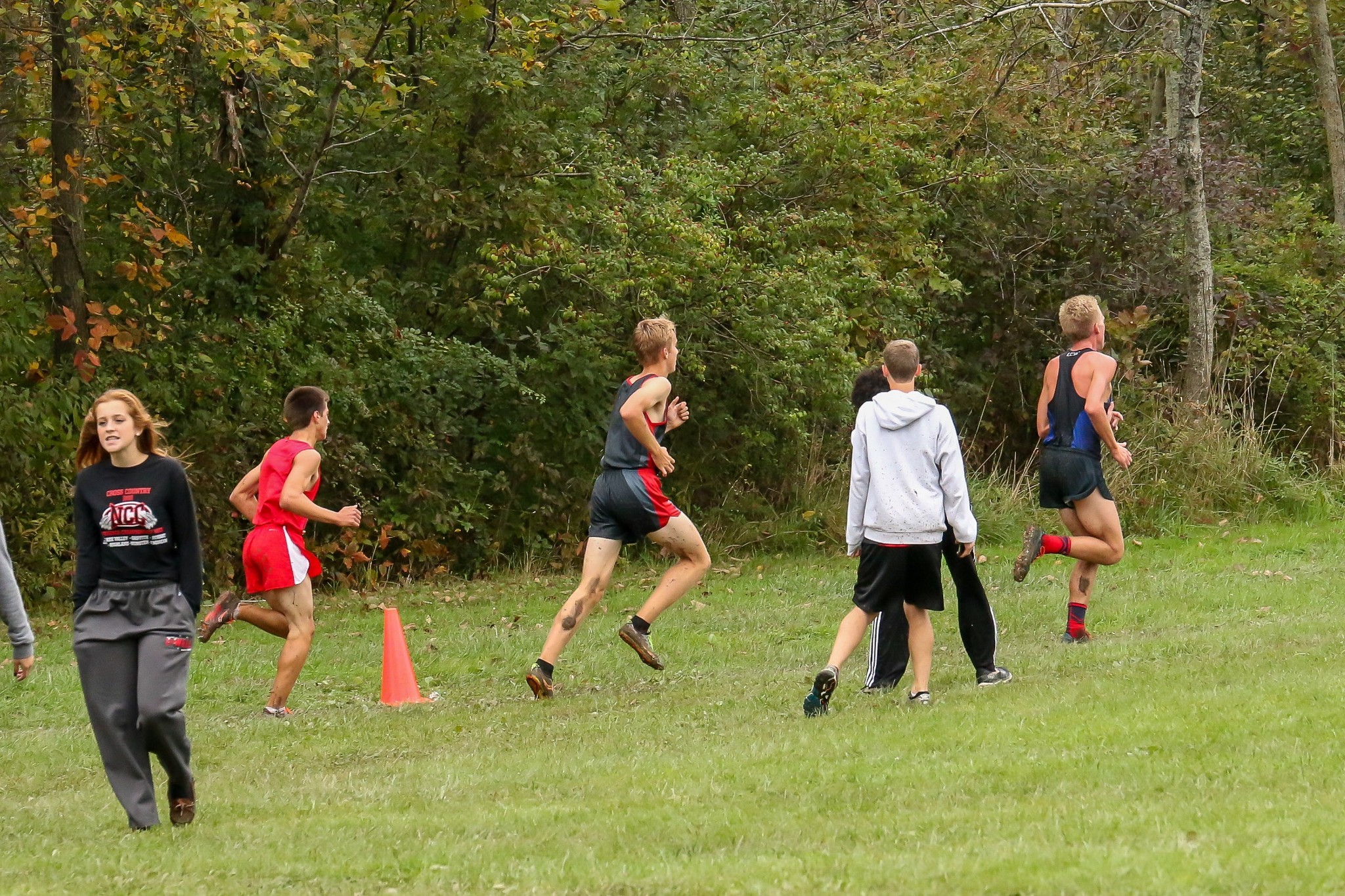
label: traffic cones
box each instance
[377,608,439,708]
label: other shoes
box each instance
[167,779,196,826]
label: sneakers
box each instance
[803,665,838,717]
[1013,525,1045,582]
[196,591,240,642]
[977,666,1011,687]
[857,686,889,694]
[617,618,665,670]
[1061,632,1097,644]
[908,691,930,705]
[525,664,555,700]
[262,707,292,720]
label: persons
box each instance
[524,318,716,699]
[202,386,364,722]
[803,341,1014,717]
[1012,293,1134,644]
[72,387,207,836]
[1,523,33,682]
